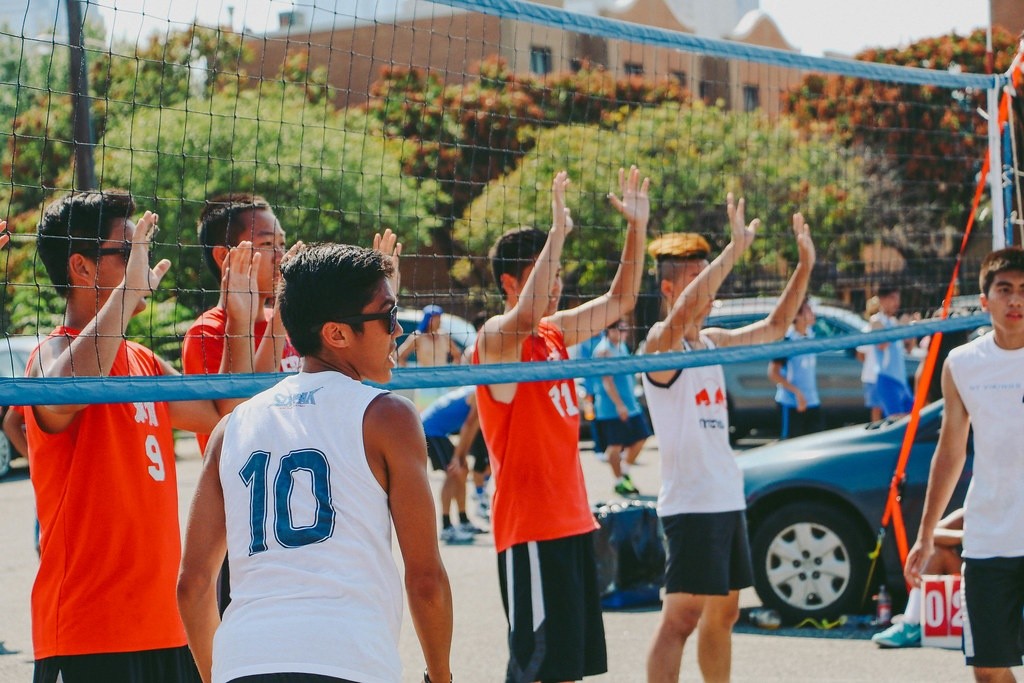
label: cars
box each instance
[730,392,976,622]
[698,296,891,445]
[1,336,48,483]
[394,309,477,364]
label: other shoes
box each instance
[471,491,489,504]
[595,452,603,461]
[440,528,475,544]
[459,523,489,534]
[477,502,490,519]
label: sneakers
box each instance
[872,615,922,647]
[614,474,640,500]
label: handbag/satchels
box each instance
[591,504,664,612]
[920,572,964,650]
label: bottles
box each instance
[877,585,892,624]
[748,607,781,629]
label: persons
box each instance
[873,502,966,648]
[395,305,462,410]
[4,404,40,560]
[419,383,490,543]
[767,304,823,443]
[471,163,650,683]
[572,328,607,452]
[642,194,819,683]
[902,246,1024,683]
[176,242,453,683]
[183,190,402,621]
[587,319,655,501]
[856,323,885,422]
[870,280,914,421]
[468,428,493,519]
[22,187,262,683]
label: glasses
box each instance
[328,294,399,334]
[82,247,153,266]
[613,324,627,332]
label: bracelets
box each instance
[423,666,453,683]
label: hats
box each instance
[417,305,444,332]
